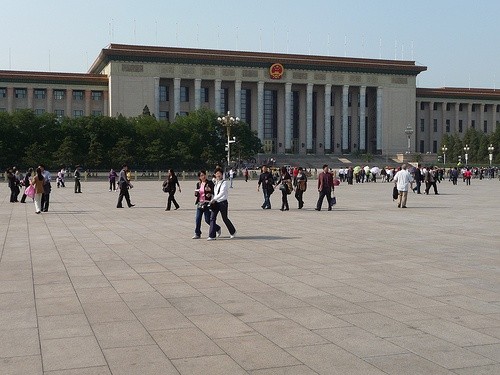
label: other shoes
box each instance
[10,199,48,214]
[328,207,332,211]
[109,190,112,192]
[315,208,320,211]
[75,192,78,193]
[206,237,216,241]
[230,231,237,239]
[398,205,401,208]
[117,206,124,208]
[129,204,135,208]
[174,207,179,210]
[261,202,304,211]
[412,189,439,195]
[78,191,82,193]
[165,209,170,211]
[402,206,407,208]
[217,228,221,237]
[192,234,200,239]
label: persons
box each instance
[207,168,237,240]
[108,169,131,191]
[192,169,221,239]
[273,166,292,211]
[392,164,413,208]
[268,165,298,186]
[257,164,275,209]
[73,165,82,193]
[309,164,500,195]
[314,164,334,210]
[4,164,67,214]
[165,168,181,210]
[213,165,235,188]
[244,168,249,182]
[293,167,307,209]
[116,164,135,208]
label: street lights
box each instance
[442,145,448,169]
[216,110,240,167]
[404,123,414,154]
[487,144,494,166]
[464,144,470,165]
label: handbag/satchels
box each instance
[393,183,398,201]
[279,184,286,190]
[163,187,169,192]
[209,201,216,211]
[24,185,35,198]
[331,197,336,206]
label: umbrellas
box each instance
[370,167,379,174]
[363,165,370,173]
[353,166,361,174]
